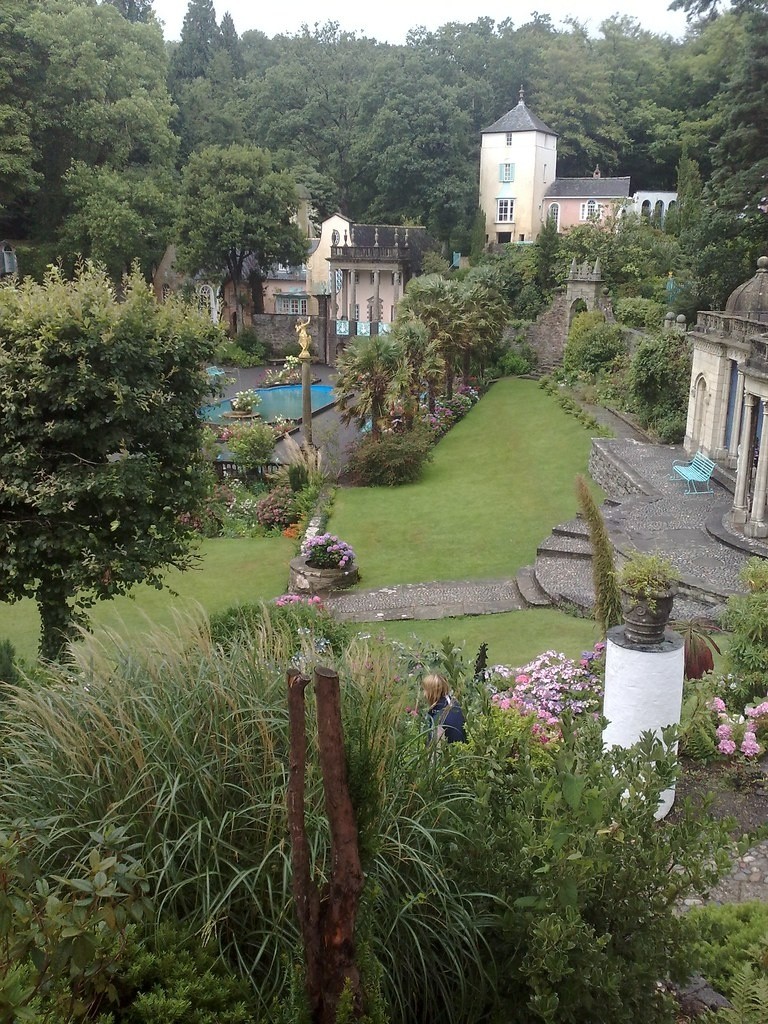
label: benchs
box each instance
[670,450,719,495]
[268,359,288,366]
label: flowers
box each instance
[301,534,357,569]
[233,387,262,412]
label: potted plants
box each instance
[606,546,680,644]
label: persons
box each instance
[295,316,312,358]
[422,674,468,744]
